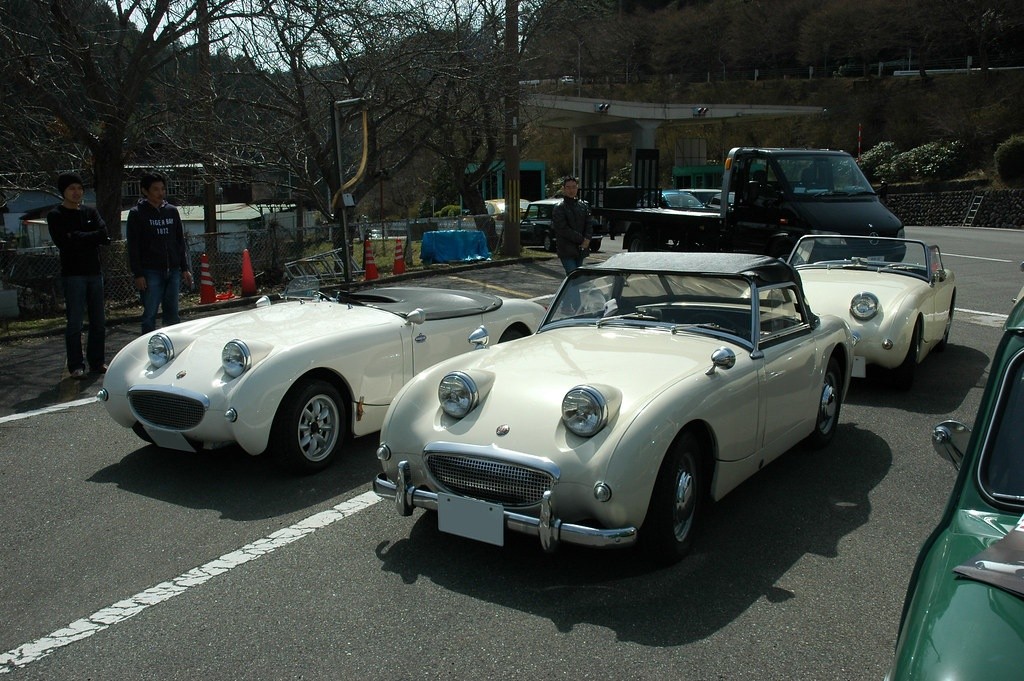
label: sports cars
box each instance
[881,258,1024,681]
[98,273,548,478]
[391,238,410,276]
[372,251,855,569]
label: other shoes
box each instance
[72,367,84,377]
[90,364,109,373]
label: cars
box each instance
[461,198,544,228]
[736,233,958,397]
[638,189,706,212]
[680,188,723,209]
[705,191,736,211]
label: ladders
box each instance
[962,195,984,227]
[282,244,366,280]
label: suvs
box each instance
[519,198,603,257]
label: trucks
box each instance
[589,144,907,264]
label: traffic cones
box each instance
[238,248,258,298]
[362,240,381,284]
[196,253,219,307]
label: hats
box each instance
[58,172,84,198]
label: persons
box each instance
[552,176,594,316]
[46,171,110,379]
[125,170,194,336]
